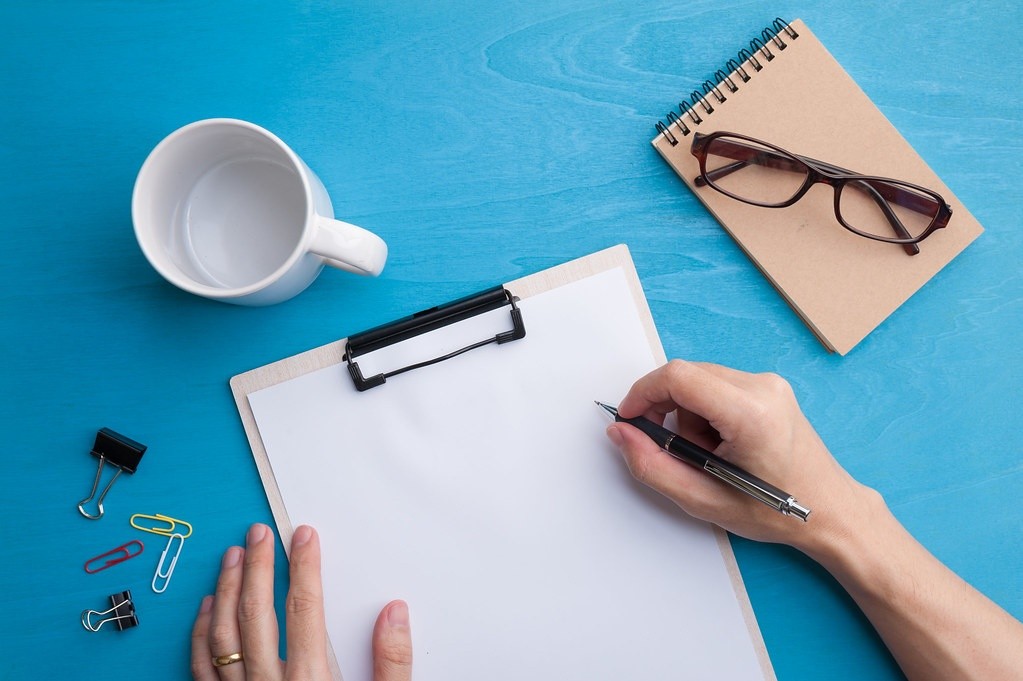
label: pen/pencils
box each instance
[593,399,813,523]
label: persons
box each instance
[191,357,1023,681]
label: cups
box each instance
[131,117,388,307]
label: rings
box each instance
[212,653,242,667]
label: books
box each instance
[650,18,987,356]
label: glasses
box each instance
[691,130,953,256]
